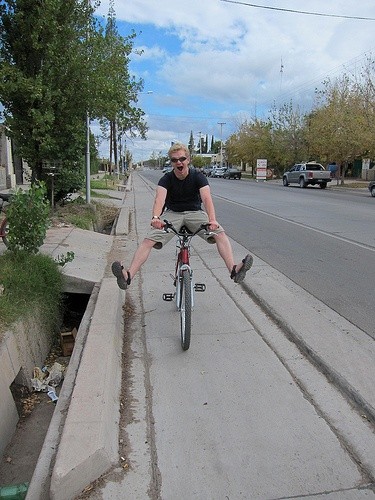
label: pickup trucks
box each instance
[281,162,333,188]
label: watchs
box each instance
[152,216,160,220]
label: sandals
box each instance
[230,254,253,283]
[111,261,131,290]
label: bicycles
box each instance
[0,192,41,252]
[160,219,219,352]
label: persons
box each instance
[111,143,254,290]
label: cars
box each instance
[224,167,240,180]
[158,165,224,177]
[368,177,375,197]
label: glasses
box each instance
[171,157,187,162]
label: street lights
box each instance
[120,90,154,178]
